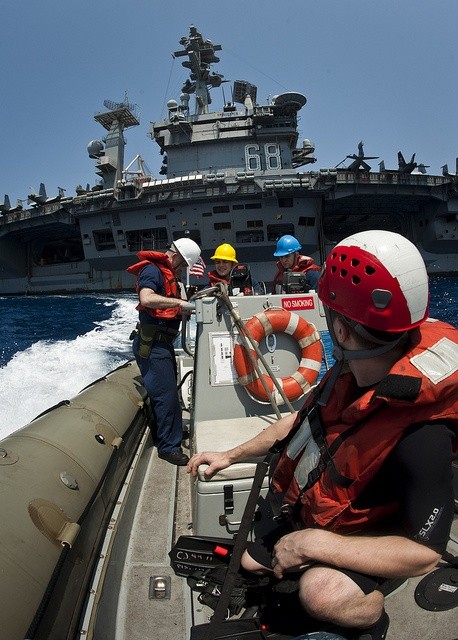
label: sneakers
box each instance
[155,431,188,443]
[158,449,189,466]
[348,610,389,639]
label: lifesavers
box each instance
[235,310,323,406]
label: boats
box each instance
[1,288,458,639]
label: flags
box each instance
[190,256,206,279]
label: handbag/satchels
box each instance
[191,617,266,640]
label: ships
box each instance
[1,21,457,296]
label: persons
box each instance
[185,227,458,639]
[271,235,317,293]
[206,244,250,296]
[133,237,198,467]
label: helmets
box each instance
[273,235,303,258]
[210,243,239,264]
[173,238,201,269]
[317,230,431,332]
